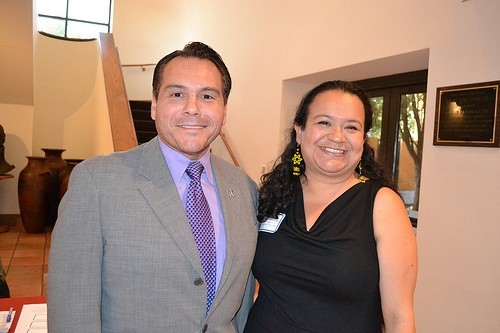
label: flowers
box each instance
[292,141,302,176]
[358,162,369,183]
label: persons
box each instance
[243,80,419,333]
[46,42,261,333]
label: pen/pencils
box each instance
[6,307,13,322]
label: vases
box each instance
[18,148,80,234]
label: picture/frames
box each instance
[433,81,500,148]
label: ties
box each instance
[185,160,217,319]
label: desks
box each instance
[0,296,46,333]
